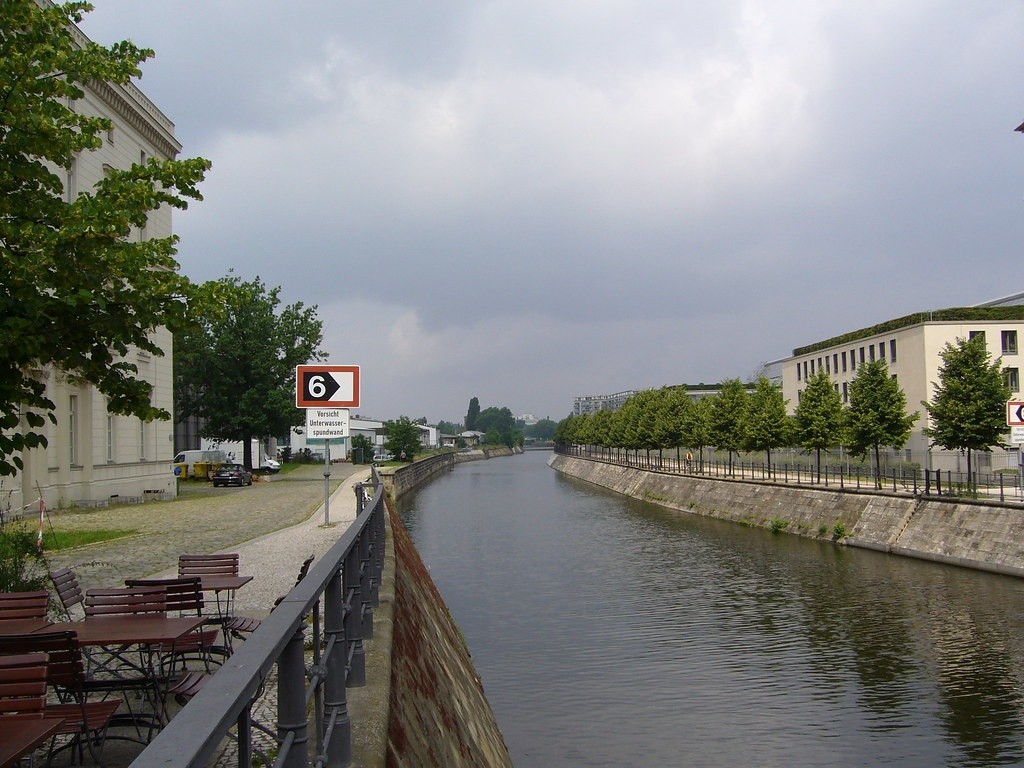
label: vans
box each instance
[174,450,226,476]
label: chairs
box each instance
[0,553,261,768]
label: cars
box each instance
[212,464,252,487]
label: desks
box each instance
[0,617,208,747]
[138,576,254,672]
[0,719,65,768]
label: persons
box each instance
[686,451,693,465]
[401,450,406,463]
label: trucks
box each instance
[201,437,281,474]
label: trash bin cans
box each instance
[193,461,213,478]
[174,463,190,478]
